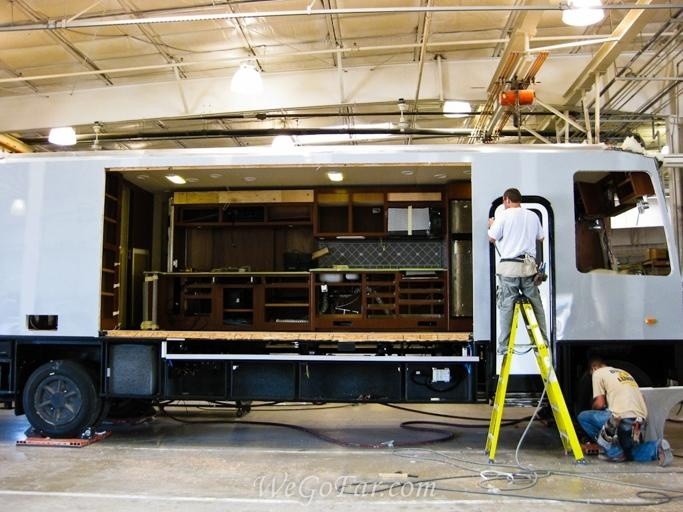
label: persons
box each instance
[578,359,674,468]
[487,189,550,355]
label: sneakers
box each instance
[597,451,627,463]
[656,437,674,468]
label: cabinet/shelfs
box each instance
[177,275,311,331]
[312,272,449,331]
[617,172,653,205]
[313,185,447,241]
[101,192,119,330]
[172,185,313,226]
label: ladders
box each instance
[484,295,586,465]
